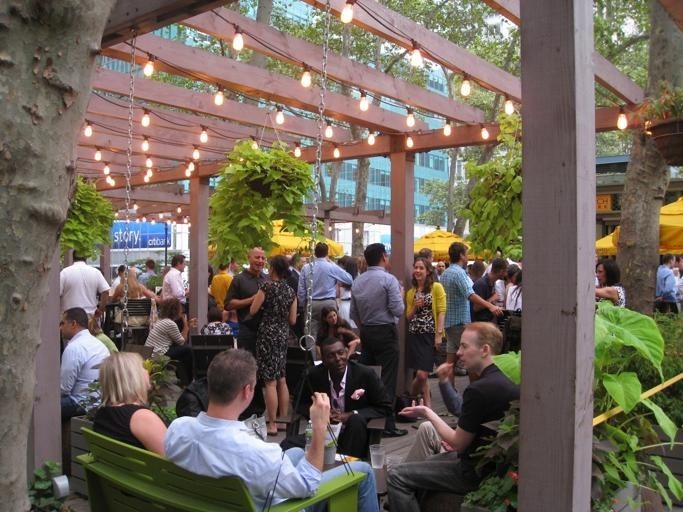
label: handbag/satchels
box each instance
[244,413,268,441]
[394,394,421,423]
[507,316,521,330]
[203,321,234,336]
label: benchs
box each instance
[62,297,518,512]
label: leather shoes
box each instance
[383,428,408,438]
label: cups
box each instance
[370,444,386,469]
[418,297,424,306]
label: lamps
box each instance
[84,118,96,139]
[477,121,491,142]
[275,103,286,126]
[212,82,226,108]
[339,0,356,25]
[299,61,314,89]
[100,162,117,188]
[294,142,303,158]
[441,116,454,138]
[404,107,417,128]
[249,136,259,152]
[404,132,415,148]
[112,197,191,228]
[230,25,245,52]
[182,143,202,178]
[408,39,424,68]
[323,118,336,140]
[365,127,376,145]
[357,89,372,114]
[139,133,152,153]
[93,146,103,162]
[459,73,473,97]
[141,155,154,185]
[139,106,153,129]
[330,143,342,159]
[502,91,515,117]
[140,52,157,77]
[197,123,210,144]
[616,104,628,130]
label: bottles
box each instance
[305,420,313,452]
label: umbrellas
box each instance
[595,232,683,256]
[612,196,683,250]
[208,218,345,258]
[414,225,493,262]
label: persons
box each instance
[59,242,683,512]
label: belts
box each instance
[312,296,335,301]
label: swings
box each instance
[75,0,368,512]
